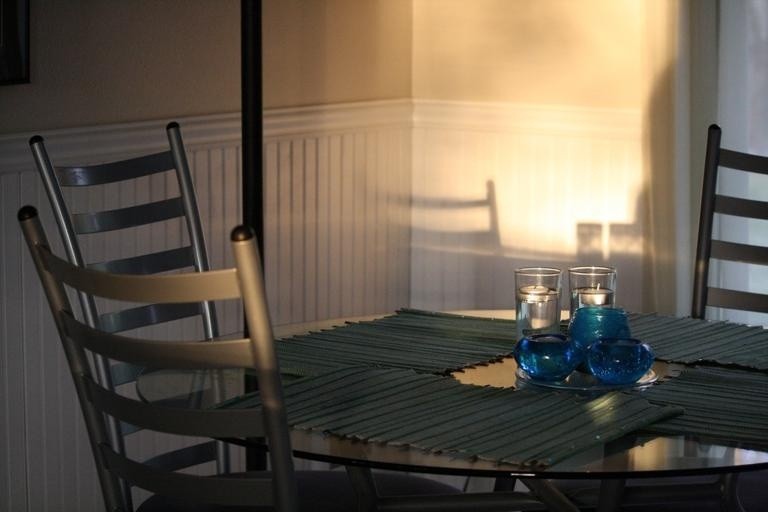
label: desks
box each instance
[135,307,768,512]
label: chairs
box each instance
[21,119,238,505]
[17,202,463,511]
[552,123,768,511]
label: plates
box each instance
[514,366,660,390]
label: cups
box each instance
[586,337,656,385]
[569,267,618,313]
[567,307,632,374]
[514,268,563,337]
[513,333,583,382]
